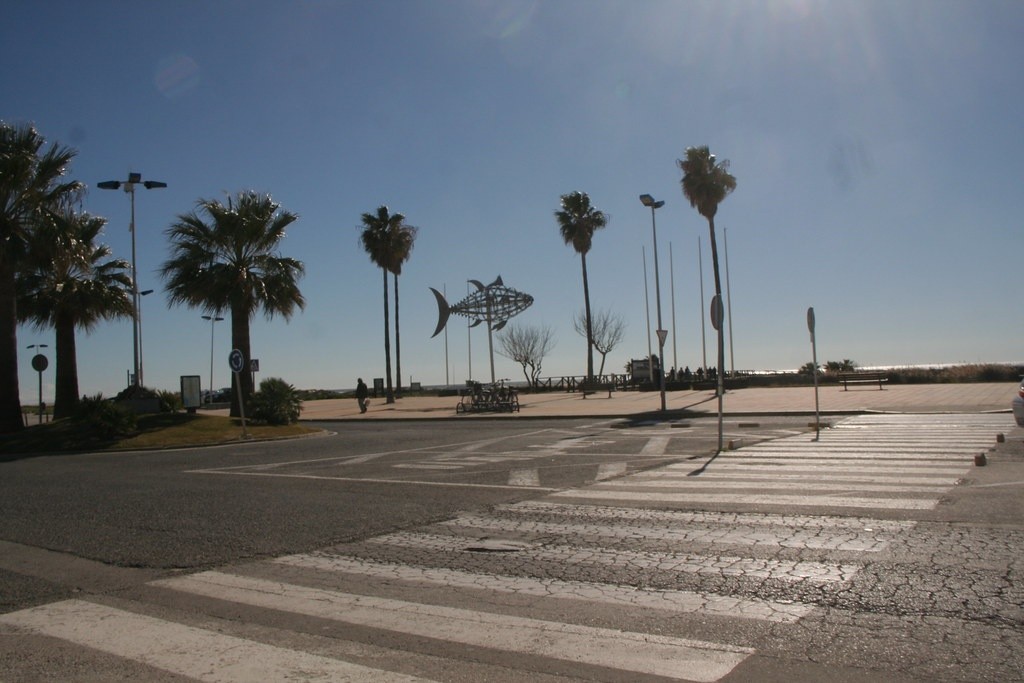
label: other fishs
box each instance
[428,275,535,339]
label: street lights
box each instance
[96,172,168,387]
[638,193,665,412]
[199,316,224,404]
[25,344,48,424]
[124,288,154,389]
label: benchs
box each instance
[581,382,617,398]
[838,371,890,391]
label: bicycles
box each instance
[456,377,519,412]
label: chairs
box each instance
[456,385,519,414]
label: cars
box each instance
[204,387,232,404]
[1012,378,1024,428]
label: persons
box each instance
[669,366,717,381]
[355,378,367,414]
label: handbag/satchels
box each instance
[363,398,370,406]
